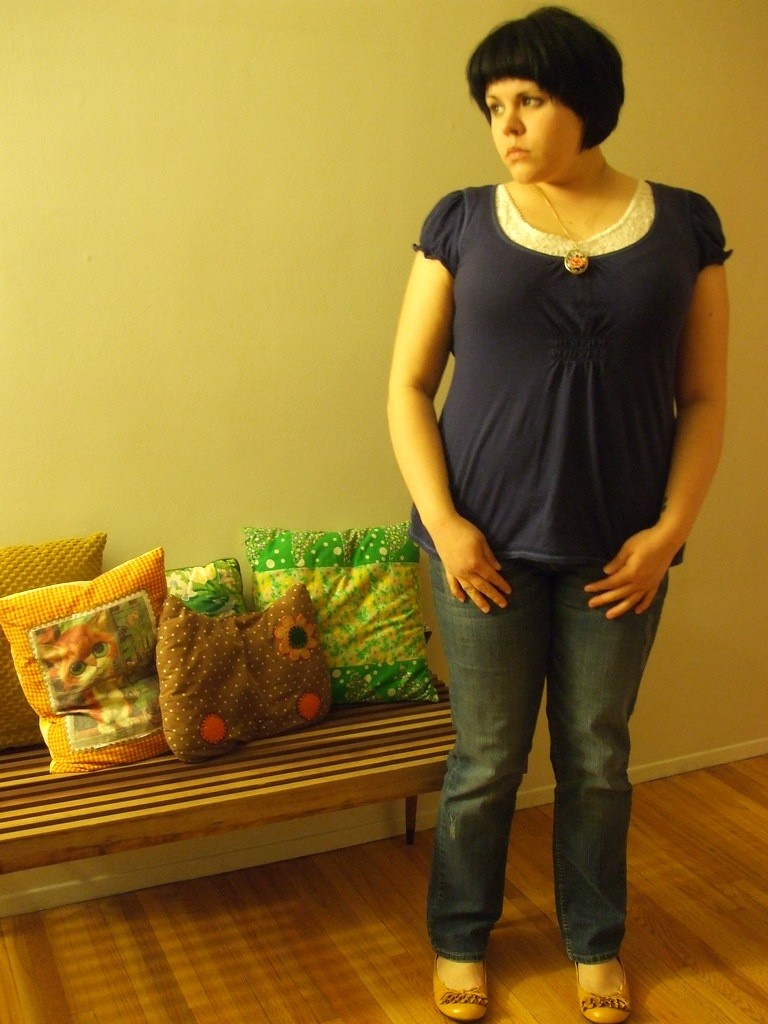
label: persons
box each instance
[386,5,731,1024]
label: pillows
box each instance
[164,558,247,622]
[241,520,440,703]
[0,533,107,749]
[155,583,333,763]
[0,546,171,772]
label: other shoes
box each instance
[575,955,631,1024]
[433,953,488,1024]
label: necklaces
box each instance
[531,155,607,276]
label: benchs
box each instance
[0,672,458,873]
